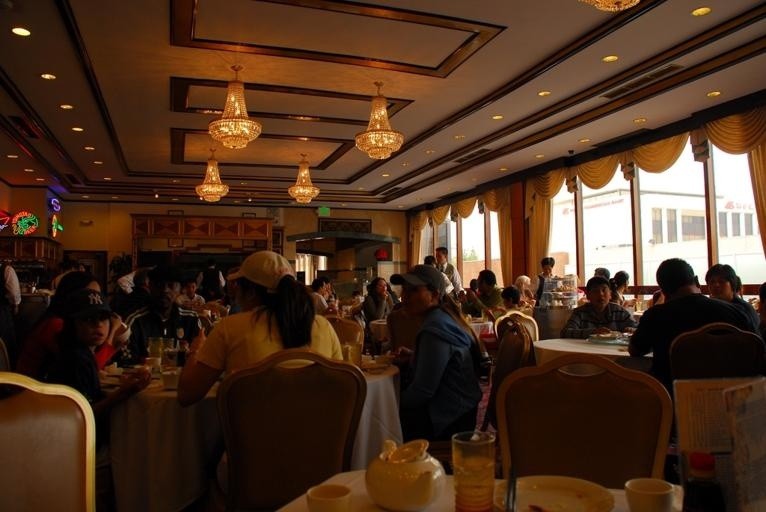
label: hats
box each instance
[390,265,445,296]
[151,259,184,282]
[67,289,113,318]
[227,251,296,293]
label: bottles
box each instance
[31,282,36,294]
[148,335,162,357]
[177,340,187,367]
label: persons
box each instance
[0,248,766,512]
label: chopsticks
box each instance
[506,462,518,511]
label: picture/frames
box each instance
[318,218,372,233]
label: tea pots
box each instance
[364,440,446,511]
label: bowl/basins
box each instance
[363,364,389,374]
[374,354,396,367]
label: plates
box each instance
[493,475,615,512]
[589,333,620,341]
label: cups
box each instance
[161,371,180,390]
[452,430,498,512]
[635,301,643,312]
[202,310,211,319]
[481,310,489,322]
[349,343,362,367]
[108,367,124,375]
[624,477,675,512]
[307,484,352,512]
[145,357,162,379]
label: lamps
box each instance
[355,80,405,160]
[209,65,263,150]
[195,149,229,202]
[288,152,320,204]
[578,0,641,12]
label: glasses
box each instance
[149,279,177,290]
